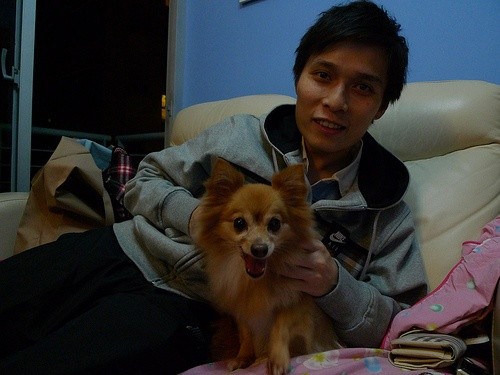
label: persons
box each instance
[0,3,426,375]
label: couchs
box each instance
[170,78,499,306]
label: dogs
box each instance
[189,156,339,375]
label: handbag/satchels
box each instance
[10,132,116,257]
